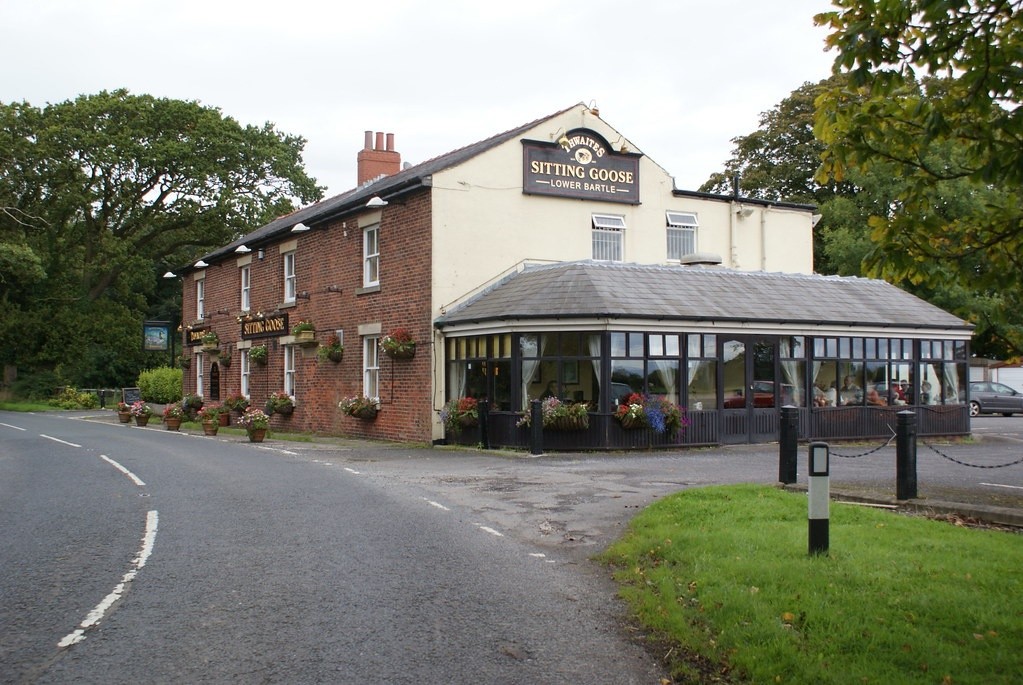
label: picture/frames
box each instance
[562,359,579,385]
[532,365,541,384]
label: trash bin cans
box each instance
[100,389,115,408]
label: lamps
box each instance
[235,245,266,254]
[236,305,267,320]
[193,260,222,267]
[582,99,599,117]
[612,134,629,154]
[737,207,755,217]
[162,271,189,278]
[291,222,329,233]
[177,320,193,333]
[366,197,406,208]
[550,127,568,146]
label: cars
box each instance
[970,381,1023,417]
[724,381,785,409]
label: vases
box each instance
[248,429,267,441]
[183,360,190,368]
[327,350,342,362]
[295,331,315,341]
[390,345,415,358]
[203,342,217,349]
[621,414,645,429]
[276,404,293,413]
[547,416,575,428]
[165,418,181,431]
[190,408,197,420]
[136,414,150,426]
[459,414,470,425]
[218,413,228,428]
[202,420,220,436]
[119,413,130,423]
[253,355,268,364]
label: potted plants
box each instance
[217,351,232,367]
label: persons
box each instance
[540,379,557,403]
[813,382,825,394]
[922,382,933,405]
[825,381,843,407]
[841,376,862,402]
[892,379,908,400]
[868,378,885,404]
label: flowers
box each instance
[290,319,315,337]
[178,356,190,366]
[437,397,478,433]
[237,406,271,440]
[201,331,218,343]
[516,395,589,429]
[338,395,378,415]
[378,328,415,355]
[247,344,268,362]
[163,395,229,431]
[317,335,344,366]
[264,391,292,413]
[117,400,154,417]
[614,392,691,441]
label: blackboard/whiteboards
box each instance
[122,388,141,404]
